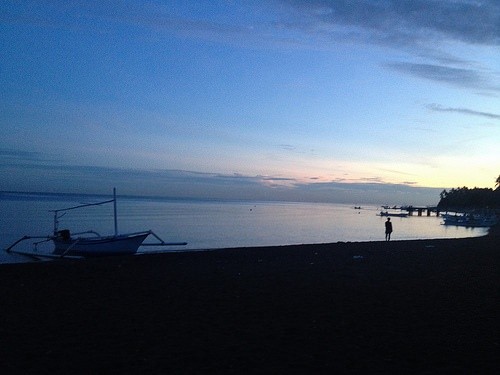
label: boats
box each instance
[3,187,188,260]
[355,207,360,209]
[381,212,408,217]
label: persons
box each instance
[385,217,392,241]
